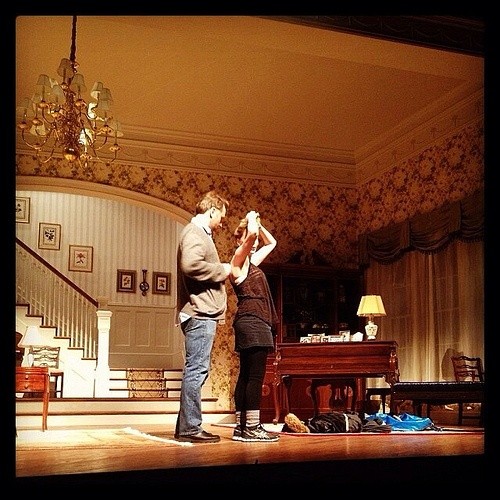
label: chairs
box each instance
[450,355,484,426]
[28,346,65,398]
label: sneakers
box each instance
[242,426,280,442]
[232,425,244,441]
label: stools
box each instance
[366,387,391,415]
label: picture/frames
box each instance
[16,196,31,223]
[38,222,61,250]
[69,245,93,272]
[117,269,137,292]
[151,272,171,294]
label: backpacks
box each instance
[368,411,442,432]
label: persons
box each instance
[174,190,241,443]
[228,211,280,442]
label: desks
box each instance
[16,364,50,431]
[274,340,401,429]
[392,378,484,427]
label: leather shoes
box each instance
[174,432,179,440]
[178,430,221,443]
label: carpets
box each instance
[212,423,474,435]
[16,427,190,450]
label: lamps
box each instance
[357,296,387,340]
[16,16,128,171]
